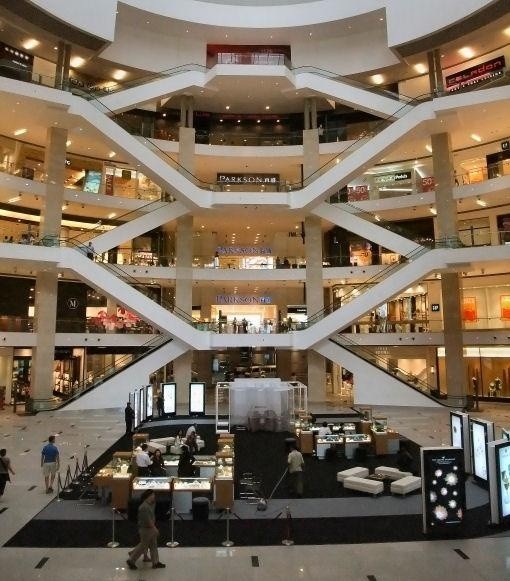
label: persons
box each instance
[242,318,248,335]
[124,402,135,437]
[374,315,381,333]
[369,313,375,333]
[177,446,197,478]
[39,435,62,496]
[285,445,306,497]
[135,443,153,477]
[2,233,40,247]
[81,242,97,262]
[185,423,201,439]
[0,449,16,502]
[149,448,169,477]
[184,432,200,454]
[316,421,333,437]
[232,318,238,335]
[128,498,158,565]
[156,392,167,420]
[173,429,189,453]
[126,488,167,571]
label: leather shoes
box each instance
[49,488,53,492]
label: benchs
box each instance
[337,466,421,498]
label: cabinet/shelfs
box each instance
[132,460,216,521]
[310,423,372,460]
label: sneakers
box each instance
[127,550,165,569]
